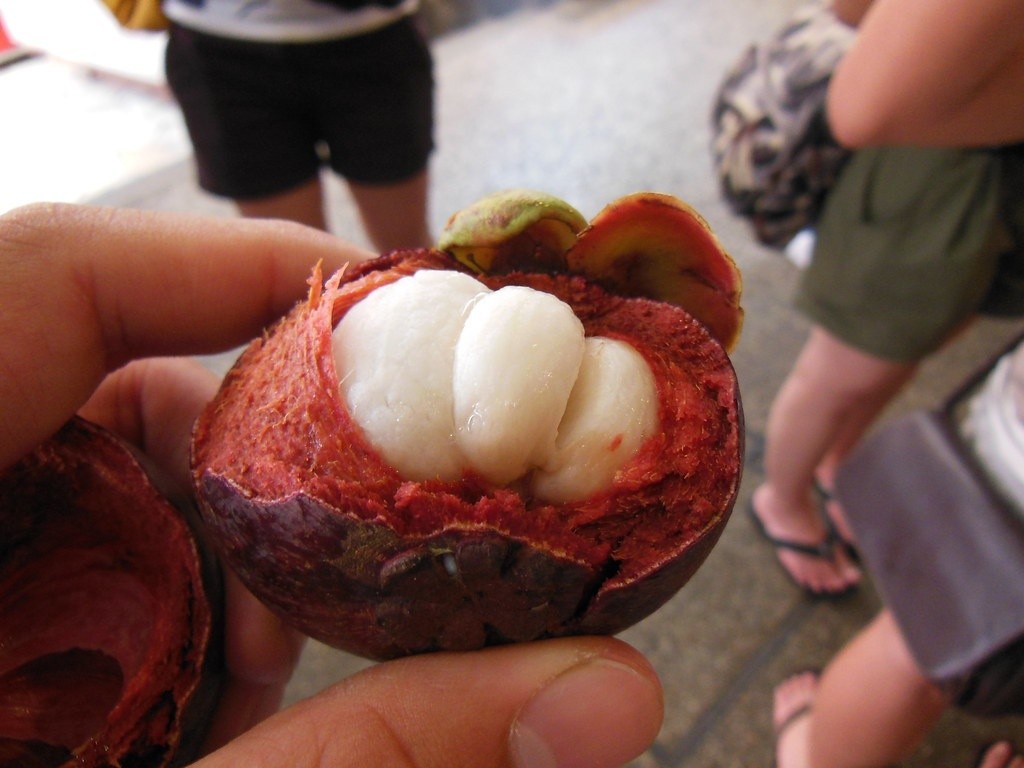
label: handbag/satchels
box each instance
[709,8,867,249]
[835,412,1024,701]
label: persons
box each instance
[154,0,441,255]
[751,0,1024,602]
[770,315,1024,767]
[0,198,670,767]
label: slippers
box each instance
[772,667,818,768]
[745,480,860,601]
